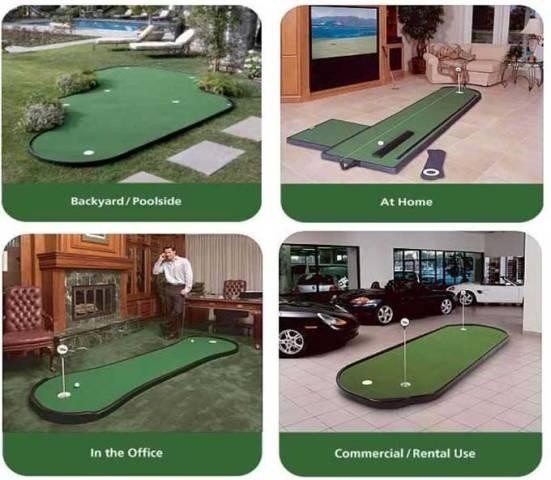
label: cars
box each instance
[278,294,357,357]
[328,278,458,328]
[395,264,474,290]
[446,274,524,308]
[295,274,337,292]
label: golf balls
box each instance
[377,141,383,145]
[190,339,194,343]
[73,382,80,389]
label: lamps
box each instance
[520,18,544,62]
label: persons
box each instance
[152,244,193,340]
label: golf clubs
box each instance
[381,46,400,90]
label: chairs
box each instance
[423,43,513,87]
[92,25,197,57]
[3,286,56,372]
[223,280,251,336]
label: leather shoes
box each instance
[160,332,177,340]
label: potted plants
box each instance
[398,6,444,74]
[507,46,524,62]
[132,5,167,40]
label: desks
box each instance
[185,297,262,350]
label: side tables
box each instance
[501,60,543,92]
